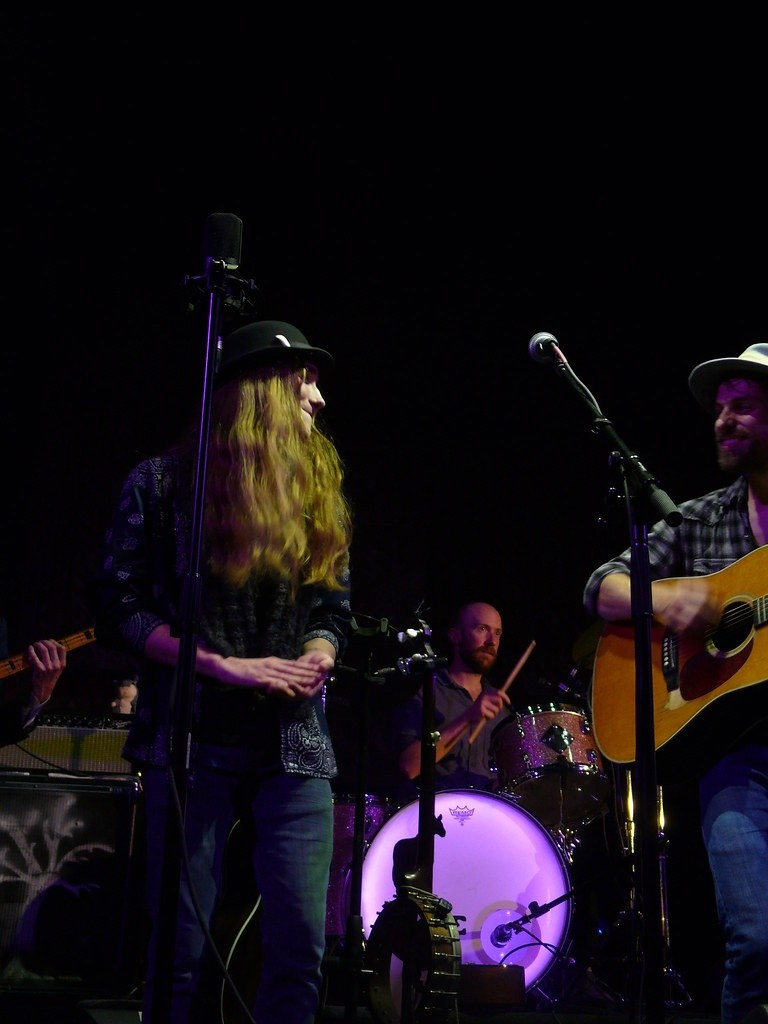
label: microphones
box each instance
[495,923,513,944]
[539,678,582,701]
[530,333,560,358]
[376,658,449,677]
[202,212,243,270]
[554,662,581,702]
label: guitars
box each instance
[0,627,96,684]
[590,532,768,764]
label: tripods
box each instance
[584,768,695,1014]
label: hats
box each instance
[212,321,333,391]
[688,343,768,414]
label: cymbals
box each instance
[355,887,460,1024]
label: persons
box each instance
[101,319,352,1022]
[391,602,512,793]
[0,620,68,748]
[109,675,140,718]
[583,341,768,1024]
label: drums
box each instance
[325,793,398,937]
[336,786,574,1008]
[484,701,615,832]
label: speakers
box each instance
[0,773,149,999]
